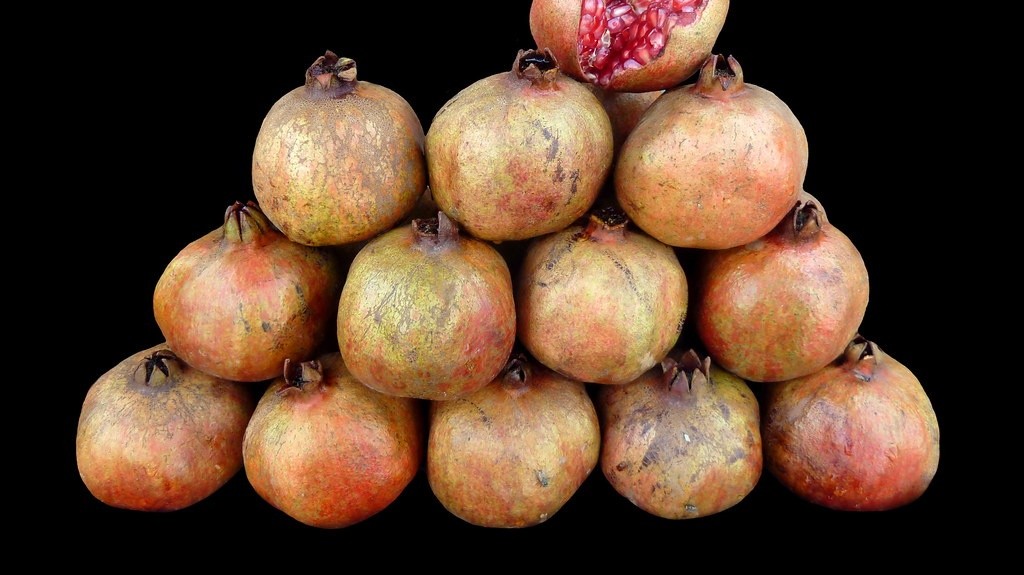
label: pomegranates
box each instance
[597,346,762,518]
[567,73,667,147]
[242,349,421,530]
[76,342,248,512]
[335,208,517,403]
[689,189,869,382]
[425,350,602,530]
[251,49,427,246]
[425,46,616,244]
[759,335,939,514]
[524,0,731,95]
[511,205,688,382]
[150,197,341,383]
[611,51,810,252]
